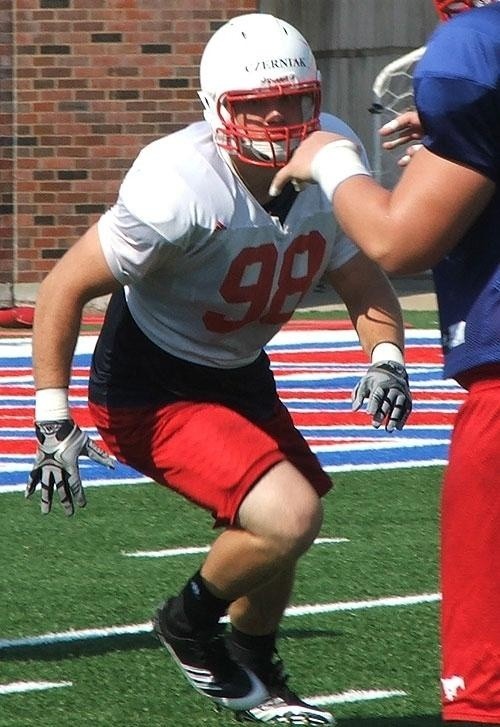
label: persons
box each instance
[21,13,414,727]
[264,2,500,725]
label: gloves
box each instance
[24,388,116,517]
[351,341,413,433]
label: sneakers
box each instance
[151,596,337,727]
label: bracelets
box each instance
[305,140,374,198]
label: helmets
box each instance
[196,12,322,157]
